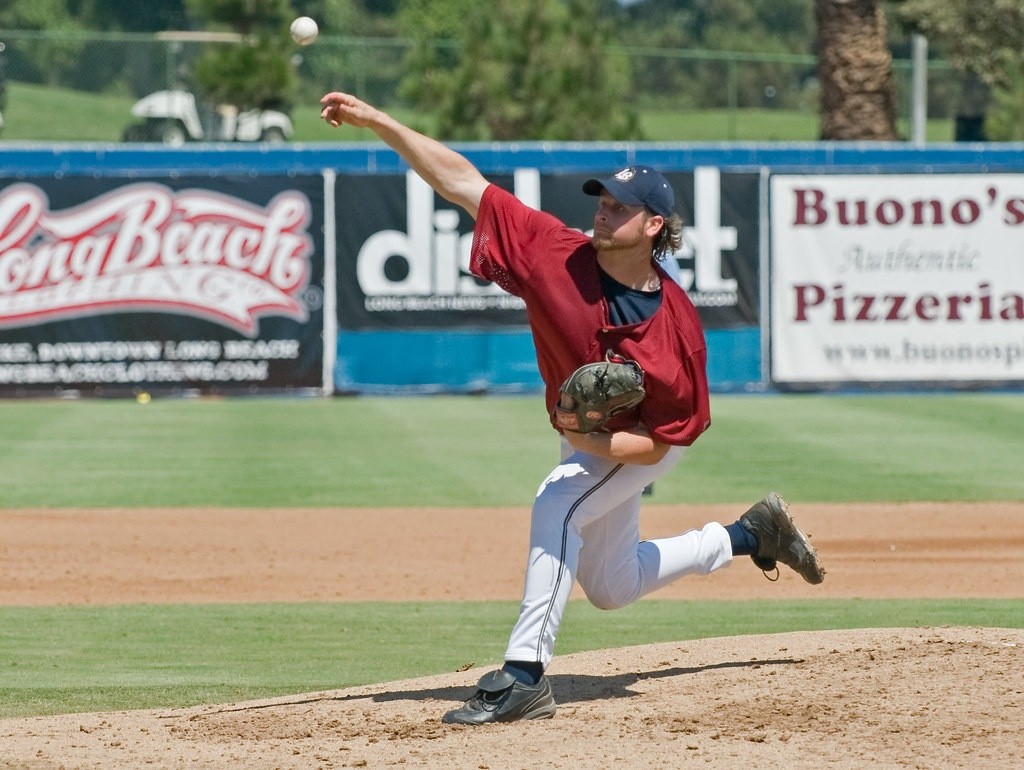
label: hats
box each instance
[584,166,674,218]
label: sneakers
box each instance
[739,492,827,585]
[443,669,556,725]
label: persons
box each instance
[954,67,990,142]
[321,91,823,725]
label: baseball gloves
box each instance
[549,361,649,433]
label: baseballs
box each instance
[289,15,318,45]
